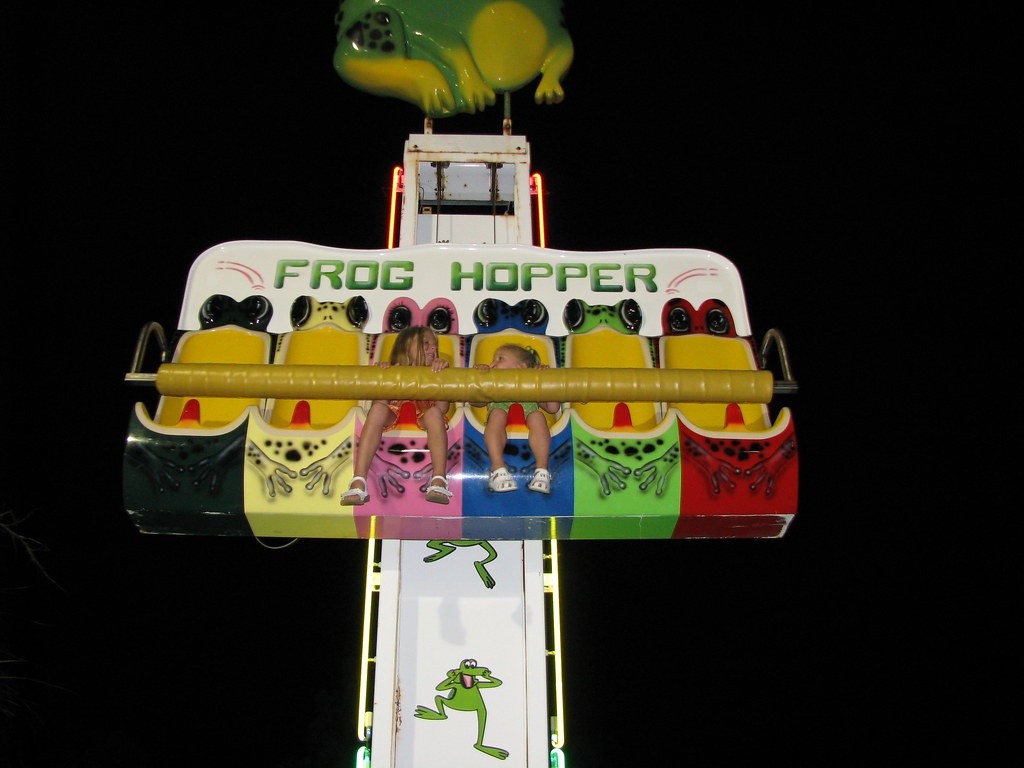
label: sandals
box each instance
[425,476,453,505]
[489,468,518,492]
[341,476,370,506]
[528,468,553,493]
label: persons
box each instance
[467,343,561,494]
[339,324,454,507]
